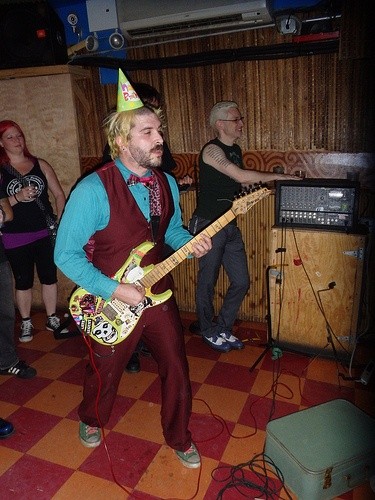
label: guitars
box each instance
[67,183,274,348]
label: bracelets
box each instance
[0,208,6,222]
[13,194,20,203]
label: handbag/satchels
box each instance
[49,222,58,242]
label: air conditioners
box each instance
[116,0,274,40]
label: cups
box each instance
[273,166,284,174]
[294,170,306,179]
[347,171,359,181]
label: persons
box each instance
[54,67,212,469]
[189,101,303,352]
[64,83,193,373]
[0,199,37,438]
[0,119,69,342]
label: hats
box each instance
[116,68,144,113]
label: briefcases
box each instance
[263,399,375,500]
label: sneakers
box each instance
[203,332,245,353]
[0,360,37,378]
[45,313,70,333]
[173,443,201,469]
[18,317,35,342]
[79,419,102,448]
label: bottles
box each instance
[27,181,37,198]
[50,225,57,240]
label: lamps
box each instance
[84,0,124,51]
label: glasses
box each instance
[220,117,244,124]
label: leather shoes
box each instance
[0,418,16,439]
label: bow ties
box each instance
[127,174,156,192]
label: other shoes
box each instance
[126,344,152,373]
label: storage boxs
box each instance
[263,402,375,499]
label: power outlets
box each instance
[281,19,298,35]
